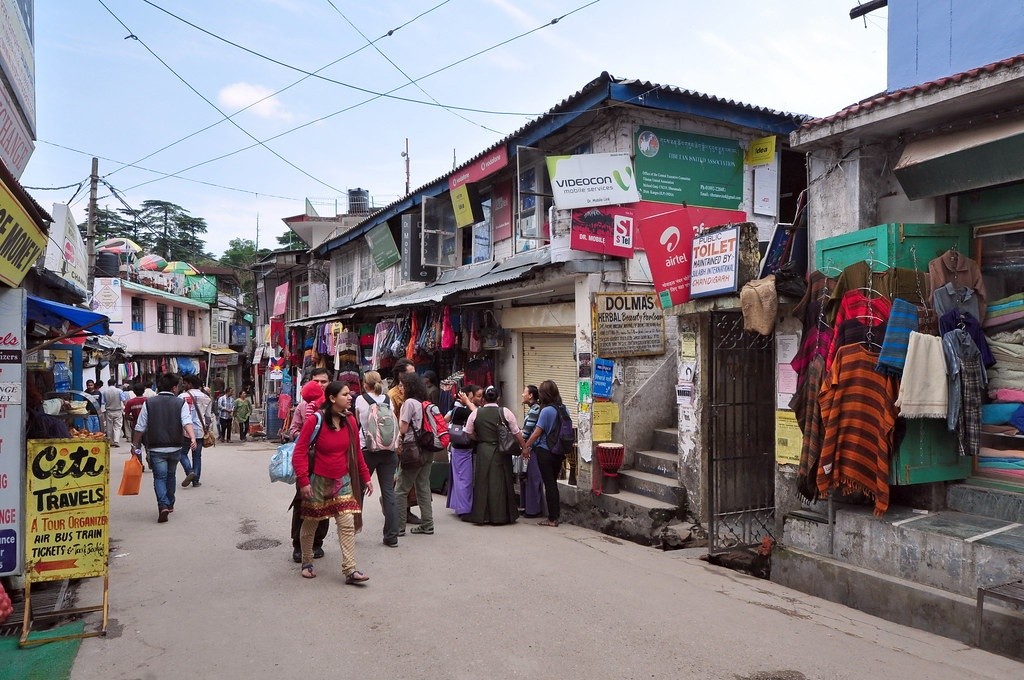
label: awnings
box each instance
[893,114,1024,202]
[201,348,237,355]
[26,294,115,356]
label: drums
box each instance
[596,442,623,494]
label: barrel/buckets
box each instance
[75,415,100,432]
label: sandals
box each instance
[537,520,559,527]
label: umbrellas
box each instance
[96,237,200,293]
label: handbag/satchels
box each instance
[448,407,472,447]
[203,431,215,448]
[377,306,505,359]
[268,412,322,485]
[117,453,142,495]
[400,442,426,470]
[496,406,520,453]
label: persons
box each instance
[287,359,434,583]
[123,383,152,473]
[168,280,175,294]
[198,378,210,397]
[214,387,252,442]
[443,379,571,526]
[177,373,211,487]
[158,274,164,284]
[173,277,178,287]
[131,372,196,523]
[102,378,127,447]
[203,387,219,430]
[83,379,104,432]
[122,377,157,443]
[25,398,74,438]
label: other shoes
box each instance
[346,571,369,584]
[398,529,406,535]
[110,443,119,448]
[218,436,247,443]
[181,472,195,487]
[193,482,202,487]
[389,542,398,547]
[302,564,316,578]
[293,548,302,562]
[410,525,434,535]
[168,509,173,513]
[312,546,324,558]
[158,506,169,523]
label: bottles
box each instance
[53,361,70,391]
[134,449,142,454]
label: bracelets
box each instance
[131,443,137,448]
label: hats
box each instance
[301,380,326,408]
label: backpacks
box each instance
[542,404,576,455]
[410,397,451,452]
[362,392,400,452]
